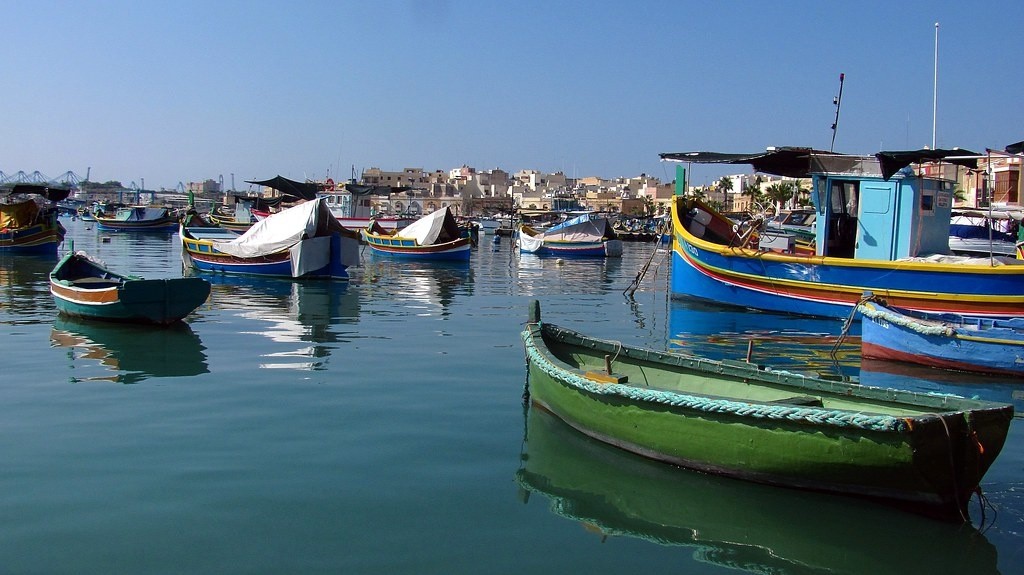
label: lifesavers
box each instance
[324,178,334,191]
[543,213,548,220]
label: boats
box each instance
[494,226,515,235]
[178,188,363,283]
[91,201,179,234]
[519,297,1016,525]
[208,194,273,235]
[363,205,472,262]
[48,238,212,329]
[514,394,1004,575]
[455,219,480,248]
[859,290,1024,380]
[664,293,865,388]
[513,212,610,257]
[335,184,427,245]
[48,312,213,384]
[658,141,1024,324]
[0,181,70,254]
